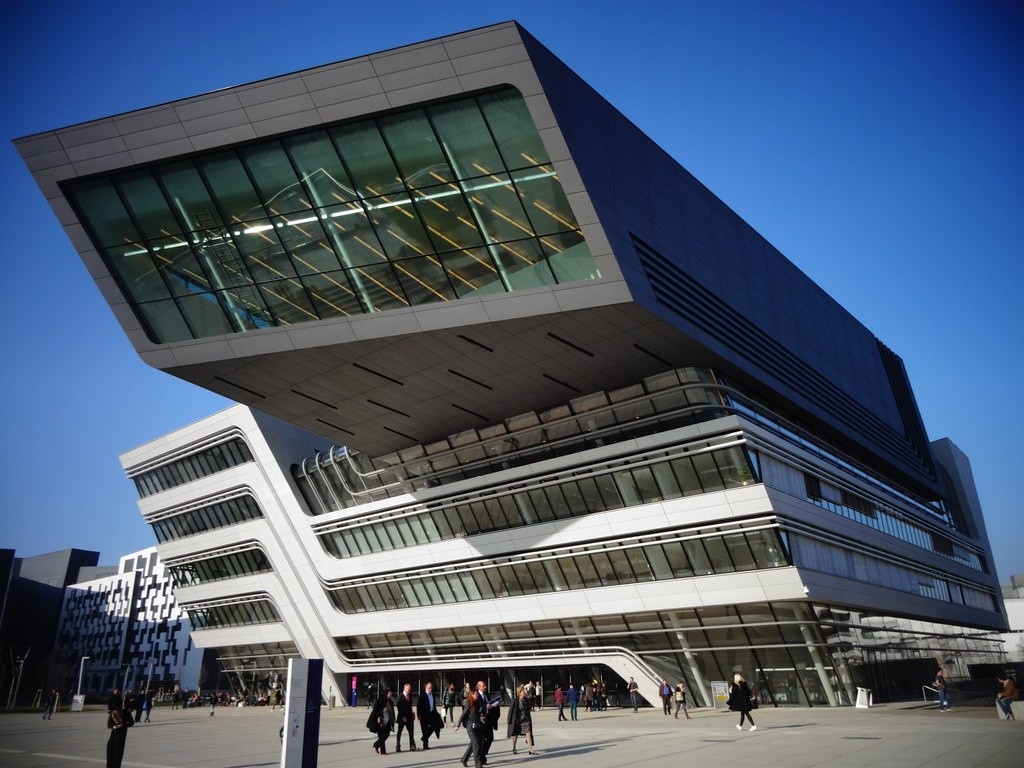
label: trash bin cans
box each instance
[854,686,873,709]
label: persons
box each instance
[172,687,284,717]
[454,680,494,768]
[43,689,56,720]
[996,673,1020,720]
[728,673,757,731]
[553,683,568,721]
[108,686,154,723]
[674,681,692,719]
[442,682,458,727]
[396,683,420,752]
[585,677,608,712]
[366,685,373,709]
[566,683,580,721]
[935,668,954,713]
[658,679,673,715]
[106,699,135,768]
[373,689,395,755]
[627,677,638,713]
[519,680,542,712]
[417,681,436,749]
[507,685,538,755]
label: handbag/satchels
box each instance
[676,692,683,700]
[750,696,758,709]
[441,708,445,716]
[526,734,534,746]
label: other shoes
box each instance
[513,748,517,754]
[749,725,757,731]
[396,745,402,752]
[529,750,540,755]
[946,709,951,712]
[381,751,389,755]
[736,724,742,730]
[373,743,379,754]
[940,710,945,712]
[410,745,416,750]
[461,760,468,767]
[1006,713,1013,720]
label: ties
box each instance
[483,693,487,703]
